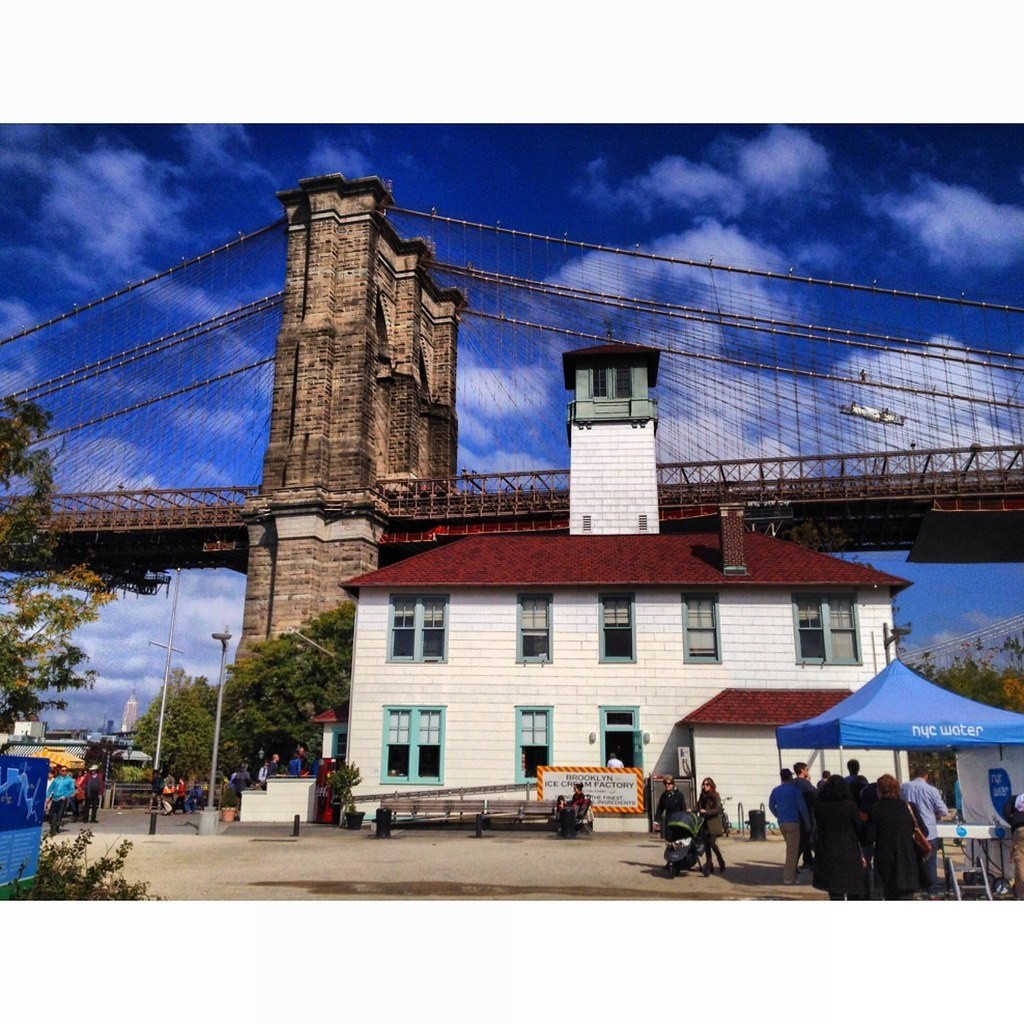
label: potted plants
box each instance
[220,787,237,823]
[326,762,366,831]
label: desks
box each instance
[935,822,1012,901]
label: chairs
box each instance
[946,856,993,901]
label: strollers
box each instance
[663,809,712,878]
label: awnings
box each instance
[99,748,152,761]
[1,745,90,759]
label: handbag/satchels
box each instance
[163,784,174,794]
[905,799,932,859]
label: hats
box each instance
[88,764,98,770]
[240,763,248,771]
[780,768,795,775]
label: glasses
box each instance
[703,782,711,787]
[62,769,68,772]
[664,781,672,785]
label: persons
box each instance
[555,783,585,834]
[607,754,625,769]
[40,753,204,837]
[653,775,687,868]
[686,778,725,878]
[769,760,1024,901]
[227,747,320,810]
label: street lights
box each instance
[198,625,232,835]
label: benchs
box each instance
[380,799,594,839]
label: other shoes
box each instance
[73,816,78,822]
[83,817,89,823]
[706,862,713,872]
[784,878,801,885]
[719,863,726,874]
[798,862,810,870]
[91,820,99,823]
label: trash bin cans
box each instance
[749,810,767,840]
[559,808,577,838]
[375,809,392,838]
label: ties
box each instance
[263,768,265,777]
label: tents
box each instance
[25,747,85,770]
[776,659,1024,880]
